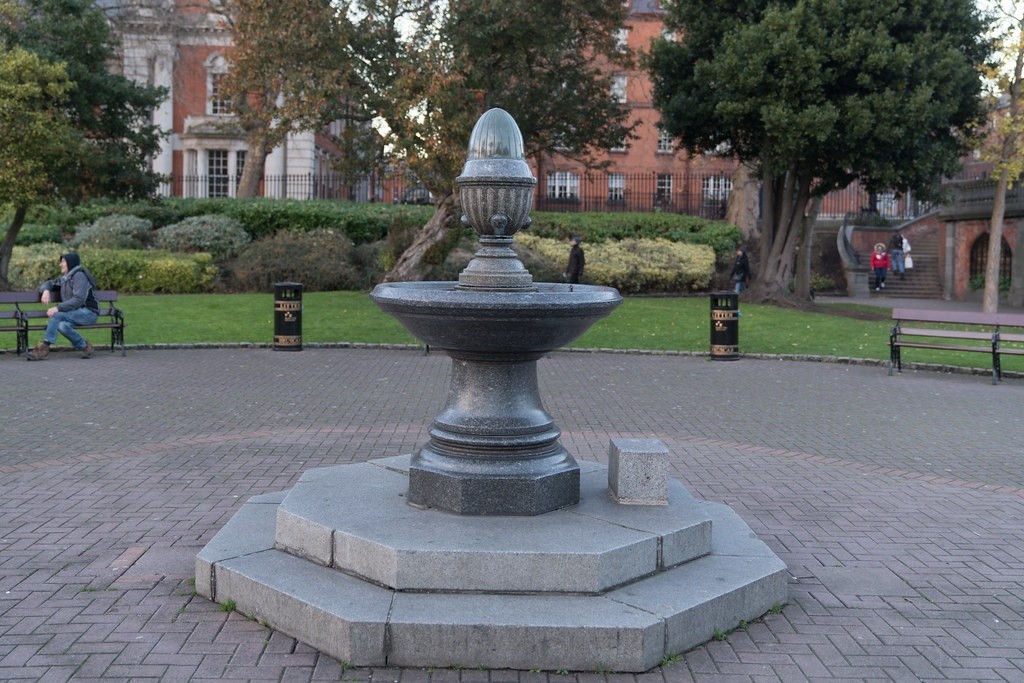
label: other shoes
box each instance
[893,270,898,275]
[81,341,95,358]
[881,282,884,288]
[875,286,880,291]
[900,273,904,280]
[26,341,51,360]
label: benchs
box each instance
[0,290,127,360]
[888,307,1024,385]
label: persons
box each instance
[889,229,905,280]
[869,242,891,292]
[26,253,100,361]
[730,249,751,294]
[564,237,585,285]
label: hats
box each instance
[574,238,582,245]
[874,243,886,251]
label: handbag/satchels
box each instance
[905,255,913,269]
[900,234,911,254]
[733,272,743,281]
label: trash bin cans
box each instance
[708,290,740,360]
[273,281,303,351]
